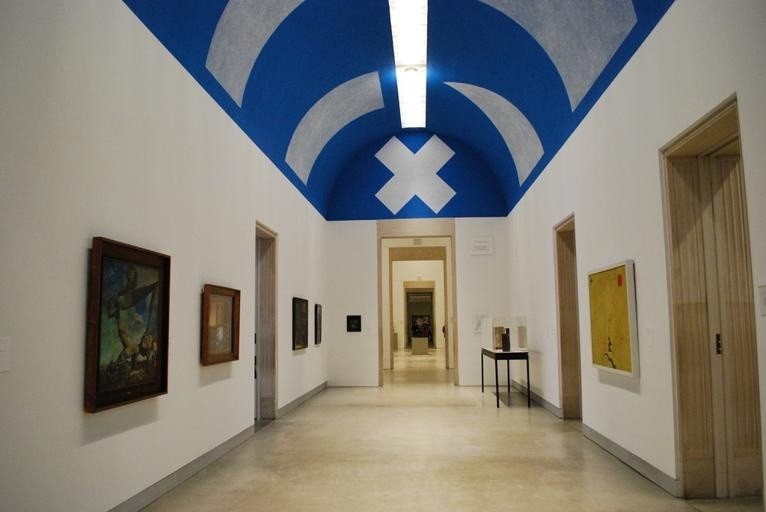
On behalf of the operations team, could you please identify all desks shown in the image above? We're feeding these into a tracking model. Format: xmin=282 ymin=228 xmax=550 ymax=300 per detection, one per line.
xmin=480 ymin=346 xmax=531 ymax=411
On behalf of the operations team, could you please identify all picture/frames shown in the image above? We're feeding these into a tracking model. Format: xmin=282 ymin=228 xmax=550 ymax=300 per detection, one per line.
xmin=314 ymin=303 xmax=322 ymax=345
xmin=291 ymin=296 xmax=309 ymax=350
xmin=347 ymin=315 xmax=361 ymax=332
xmin=83 ymin=236 xmax=171 ymax=414
xmin=586 ymin=259 xmax=641 ymax=378
xmin=200 ymin=283 xmax=242 ymax=366
